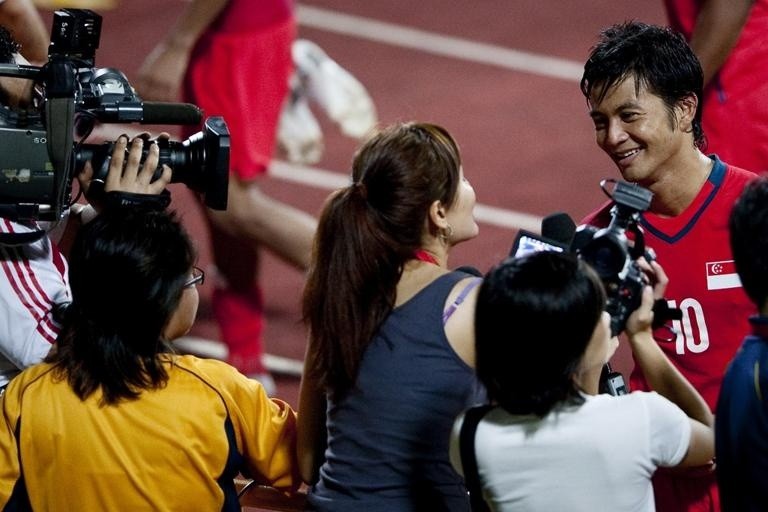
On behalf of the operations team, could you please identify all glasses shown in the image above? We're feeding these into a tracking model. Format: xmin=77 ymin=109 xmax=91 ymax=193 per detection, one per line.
xmin=182 ymin=266 xmax=205 ymax=287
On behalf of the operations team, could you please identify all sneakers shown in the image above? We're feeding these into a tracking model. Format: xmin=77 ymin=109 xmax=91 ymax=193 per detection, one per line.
xmin=276 ymin=39 xmax=377 ymax=164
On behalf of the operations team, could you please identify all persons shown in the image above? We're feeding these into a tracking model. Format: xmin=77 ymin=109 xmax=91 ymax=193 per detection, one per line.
xmin=296 ymin=121 xmax=484 ymax=512
xmin=576 ymin=1 xmax=768 ymax=512
xmin=0 ymin=0 xmax=379 ymax=510
xmin=449 ymin=244 xmax=717 ymax=511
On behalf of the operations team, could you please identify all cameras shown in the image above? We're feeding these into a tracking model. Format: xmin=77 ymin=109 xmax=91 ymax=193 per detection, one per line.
xmin=507 ymin=179 xmax=682 ymax=339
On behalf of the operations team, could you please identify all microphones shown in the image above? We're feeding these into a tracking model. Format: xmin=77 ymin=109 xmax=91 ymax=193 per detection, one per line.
xmin=538 ymin=213 xmax=576 ymax=244
